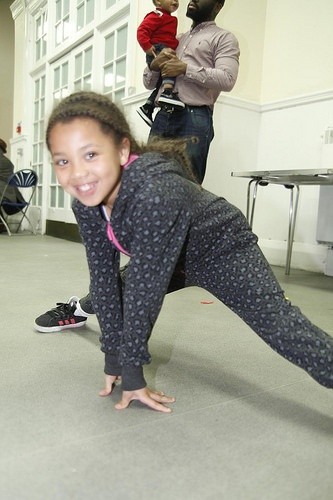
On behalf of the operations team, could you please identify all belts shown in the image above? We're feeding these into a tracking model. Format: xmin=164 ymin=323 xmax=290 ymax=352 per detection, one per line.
xmin=164 ymin=104 xmax=180 ymax=113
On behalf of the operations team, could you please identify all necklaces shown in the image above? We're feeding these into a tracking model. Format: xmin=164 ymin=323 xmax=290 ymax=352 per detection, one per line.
xmin=32 ymin=91 xmax=333 ymax=412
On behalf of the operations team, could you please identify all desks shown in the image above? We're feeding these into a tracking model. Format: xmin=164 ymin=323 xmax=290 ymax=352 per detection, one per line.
xmin=232 ymin=169 xmax=333 ymax=276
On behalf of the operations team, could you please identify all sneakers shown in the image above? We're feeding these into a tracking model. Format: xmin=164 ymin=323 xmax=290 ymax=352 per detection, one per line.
xmin=158 ymin=91 xmax=186 ymax=109
xmin=34 ymin=295 xmax=88 ymax=333
xmin=137 ymin=105 xmax=154 ymax=127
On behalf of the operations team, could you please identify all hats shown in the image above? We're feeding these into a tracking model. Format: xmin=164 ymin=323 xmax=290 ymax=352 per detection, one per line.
xmin=45 ymin=90 xmax=133 ymax=146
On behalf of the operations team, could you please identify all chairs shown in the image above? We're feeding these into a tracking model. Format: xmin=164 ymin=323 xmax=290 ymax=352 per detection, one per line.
xmin=0 ymin=169 xmax=38 ymax=236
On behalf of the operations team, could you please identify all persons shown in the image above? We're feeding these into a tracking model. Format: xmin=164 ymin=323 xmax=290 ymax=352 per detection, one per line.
xmin=0 ymin=139 xmax=25 ymax=233
xmin=141 ymin=0 xmax=240 ymax=185
xmin=136 ymin=0 xmax=185 ymax=126
